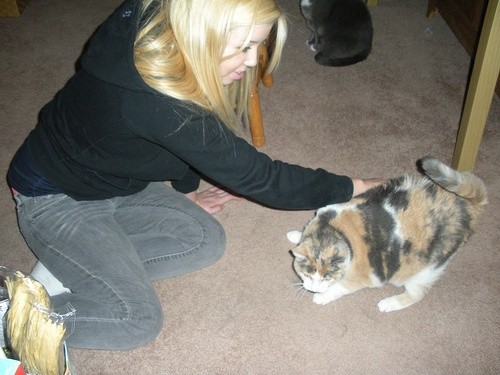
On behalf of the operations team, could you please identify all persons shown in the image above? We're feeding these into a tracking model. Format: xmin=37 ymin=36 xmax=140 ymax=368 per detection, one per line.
xmin=1 ymin=0 xmax=391 ymax=351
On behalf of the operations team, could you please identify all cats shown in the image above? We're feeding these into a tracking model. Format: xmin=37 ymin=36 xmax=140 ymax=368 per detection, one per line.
xmin=301 ymin=0 xmax=373 ymax=67
xmin=286 ymin=155 xmax=488 ymax=312
